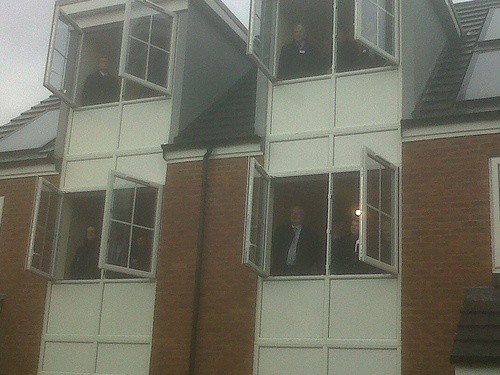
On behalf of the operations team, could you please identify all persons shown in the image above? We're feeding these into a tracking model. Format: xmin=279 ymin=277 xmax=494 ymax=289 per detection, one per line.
xmin=278 ymin=24 xmax=319 ymax=81
xmin=70 ymin=225 xmax=152 ymax=280
xmin=271 ymin=205 xmax=314 ymax=277
xmin=331 ymin=217 xmax=391 ymax=274
xmin=84 ymin=57 xmax=120 ymax=106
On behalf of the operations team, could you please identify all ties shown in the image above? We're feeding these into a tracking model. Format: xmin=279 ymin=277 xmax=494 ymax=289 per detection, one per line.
xmin=110 ymin=245 xmax=116 ymax=265
xmin=298 ymin=44 xmax=301 ymax=49
